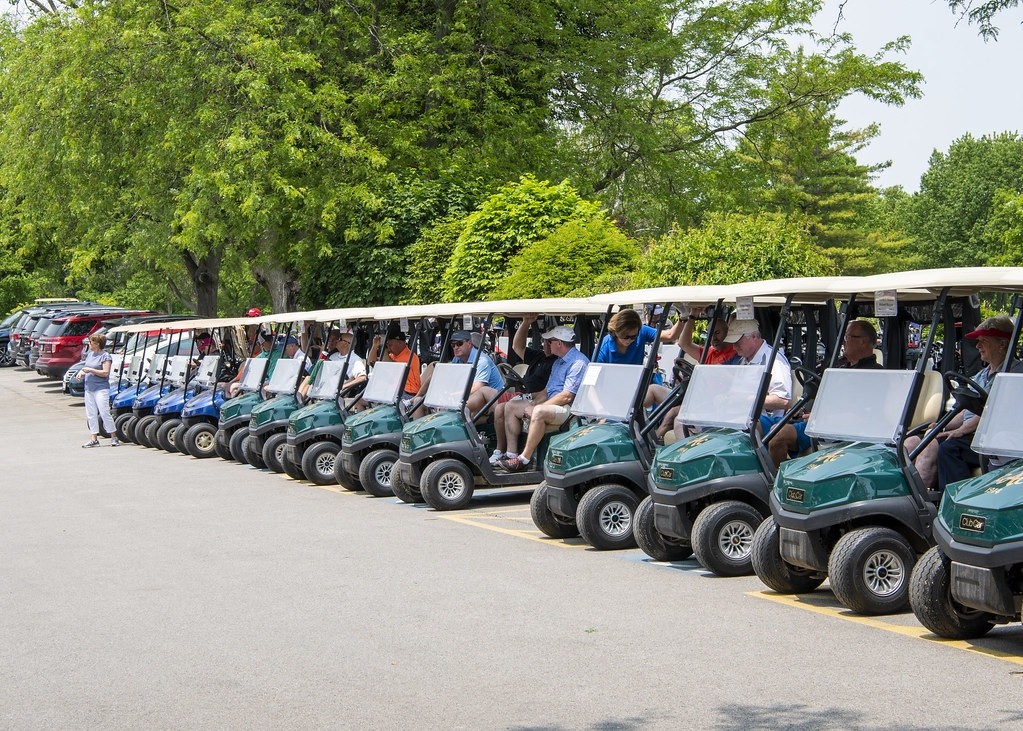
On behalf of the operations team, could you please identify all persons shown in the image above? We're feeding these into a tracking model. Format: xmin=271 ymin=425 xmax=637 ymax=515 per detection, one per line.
xmin=592 ymin=307 xmax=1022 ymax=508
xmin=495 ymin=325 xmax=591 ymax=473
xmin=190 ymin=312 xmax=558 ymax=465
xmin=75 ymin=334 xmax=120 ymax=448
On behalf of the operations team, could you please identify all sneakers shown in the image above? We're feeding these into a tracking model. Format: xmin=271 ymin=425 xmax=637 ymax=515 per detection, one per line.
xmin=495 ymin=455 xmax=510 ymax=461
xmin=489 ymin=449 xmax=503 ymax=464
xmin=111 ymin=437 xmax=120 ymax=446
xmin=82 ymin=440 xmax=100 ymax=447
xmin=498 ymin=457 xmax=531 ymax=473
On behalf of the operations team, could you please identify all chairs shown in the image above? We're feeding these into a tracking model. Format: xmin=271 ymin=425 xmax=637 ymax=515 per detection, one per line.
xmin=910 ymin=369 xmax=943 ymax=430
xmin=788 ymin=370 xmax=808 ymax=415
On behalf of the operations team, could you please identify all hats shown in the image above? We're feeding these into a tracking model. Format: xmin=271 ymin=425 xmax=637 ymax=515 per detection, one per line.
xmin=448 ymin=331 xmax=471 ymax=341
xmin=276 ymin=336 xmax=298 ymax=348
xmin=258 ymin=331 xmax=274 ymax=346
xmin=542 ymin=326 xmax=576 ymax=342
xmin=387 ymin=331 xmax=407 ymax=341
xmin=246 ymin=307 xmax=262 ymax=316
xmin=195 ymin=332 xmax=210 ymax=343
xmin=965 ymin=325 xmax=1012 ymax=339
xmin=722 ymin=318 xmax=759 ymax=343
xmin=197 ymin=338 xmax=215 ymax=350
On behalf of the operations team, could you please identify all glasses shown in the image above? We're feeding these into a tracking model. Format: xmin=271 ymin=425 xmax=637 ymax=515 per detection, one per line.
xmin=338 ymin=337 xmax=351 ymax=345
xmin=548 ymin=339 xmax=560 ymax=344
xmin=621 ymin=330 xmax=640 ymax=341
xmin=844 ymin=335 xmax=863 ymax=341
xmin=450 ymin=340 xmax=468 ymax=347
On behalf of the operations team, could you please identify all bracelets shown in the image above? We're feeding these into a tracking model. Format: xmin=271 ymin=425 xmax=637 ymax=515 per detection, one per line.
xmin=679 ymin=318 xmax=688 ymax=323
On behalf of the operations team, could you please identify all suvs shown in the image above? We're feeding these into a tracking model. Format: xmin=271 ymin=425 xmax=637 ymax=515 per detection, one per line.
xmin=0 ymin=297 xmax=207 ymax=398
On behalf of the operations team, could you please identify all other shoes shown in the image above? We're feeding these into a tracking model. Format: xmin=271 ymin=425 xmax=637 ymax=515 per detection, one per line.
xmin=402 ymin=399 xmax=412 ymax=408
